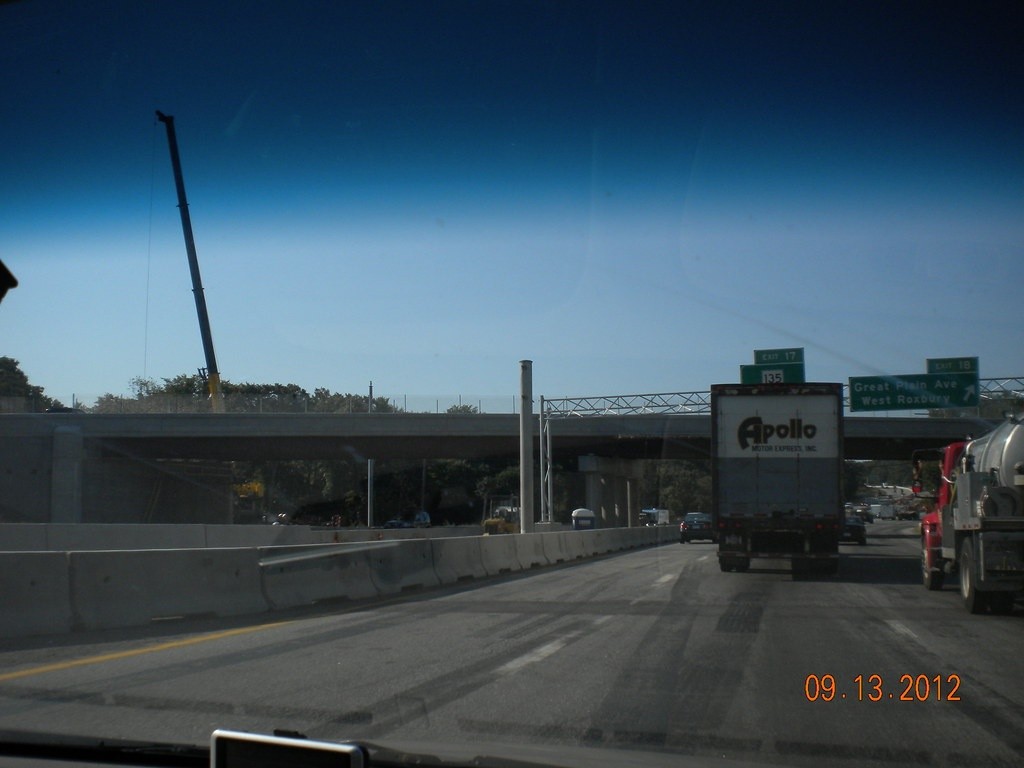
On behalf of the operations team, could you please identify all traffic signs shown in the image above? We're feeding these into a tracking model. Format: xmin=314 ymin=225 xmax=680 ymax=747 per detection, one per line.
xmin=848 ymin=355 xmax=982 ymax=413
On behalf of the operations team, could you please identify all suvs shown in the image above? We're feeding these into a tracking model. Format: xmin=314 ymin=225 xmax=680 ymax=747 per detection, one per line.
xmin=384 ymin=509 xmax=431 ymax=530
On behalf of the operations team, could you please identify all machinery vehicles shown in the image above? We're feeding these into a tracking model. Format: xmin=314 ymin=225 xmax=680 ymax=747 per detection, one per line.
xmin=153 ymin=107 xmax=266 ymax=529
xmin=480 ymin=491 xmax=520 ymax=535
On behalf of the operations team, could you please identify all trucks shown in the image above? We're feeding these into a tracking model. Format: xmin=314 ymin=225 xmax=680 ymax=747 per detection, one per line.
xmin=906 ymin=411 xmax=1024 ymax=617
xmin=708 ymin=380 xmax=848 ymax=578
xmin=638 ymin=507 xmax=670 ymax=527
xmin=571 ymin=508 xmax=595 ymax=531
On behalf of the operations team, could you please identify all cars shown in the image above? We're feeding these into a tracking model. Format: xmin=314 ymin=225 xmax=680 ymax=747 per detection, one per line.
xmin=43 ymin=407 xmax=86 ymax=414
xmin=839 ymin=491 xmax=931 ymax=546
xmin=679 ymin=512 xmax=720 ymax=546
